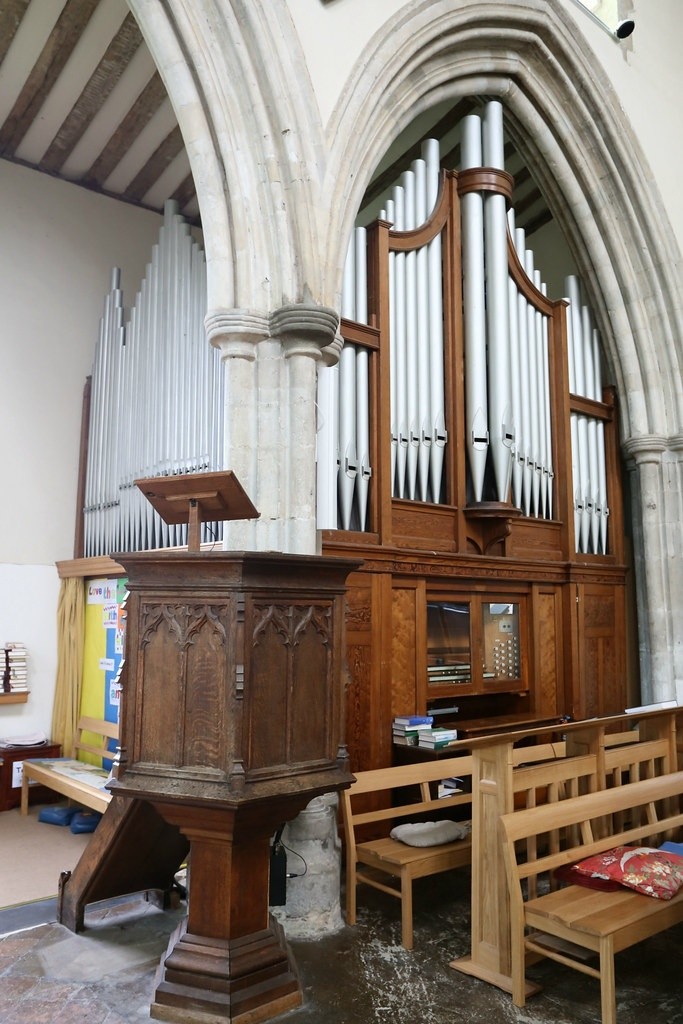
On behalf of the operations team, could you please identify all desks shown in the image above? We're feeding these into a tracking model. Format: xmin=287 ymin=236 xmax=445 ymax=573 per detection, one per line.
xmin=0 ymin=738 xmax=62 ymax=812
xmin=390 ymin=711 xmax=563 ymax=838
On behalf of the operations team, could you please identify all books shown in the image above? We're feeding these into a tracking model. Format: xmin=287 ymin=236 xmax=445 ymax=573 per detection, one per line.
xmin=392 ymin=716 xmax=433 ymax=746
xmin=441 ymin=779 xmax=461 ymax=788
xmin=417 ymin=727 xmax=457 ymax=750
xmin=4 ymin=642 xmax=31 ymax=692
xmin=0 ymin=649 xmax=7 ymax=693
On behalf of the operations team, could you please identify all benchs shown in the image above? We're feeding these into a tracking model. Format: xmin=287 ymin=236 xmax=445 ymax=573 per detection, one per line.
xmin=20 ymin=715 xmax=120 ymax=818
xmin=341 ymin=727 xmax=683 ymax=1024
